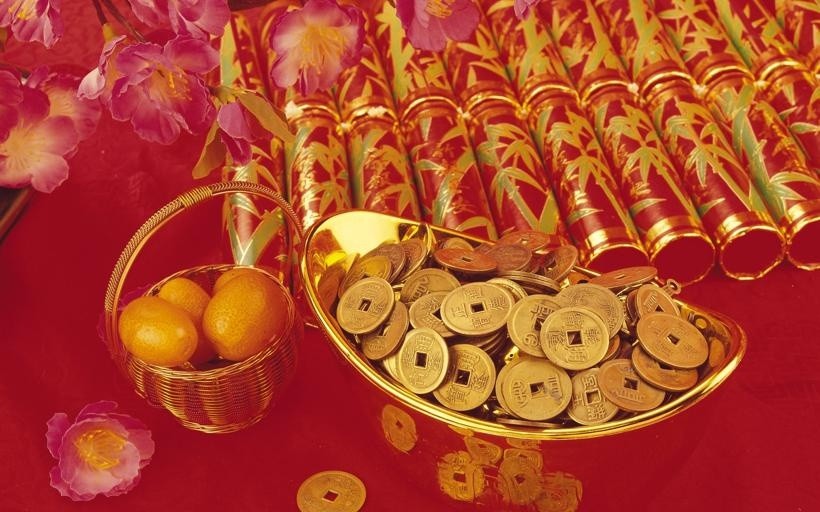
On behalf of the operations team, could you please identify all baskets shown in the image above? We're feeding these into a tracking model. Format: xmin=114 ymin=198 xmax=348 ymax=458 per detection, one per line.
xmin=104 ymin=181 xmax=304 ymax=435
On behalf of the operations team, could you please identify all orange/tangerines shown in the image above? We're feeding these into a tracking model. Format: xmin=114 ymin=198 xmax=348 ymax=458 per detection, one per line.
xmin=118 ymin=269 xmax=288 ymax=368
